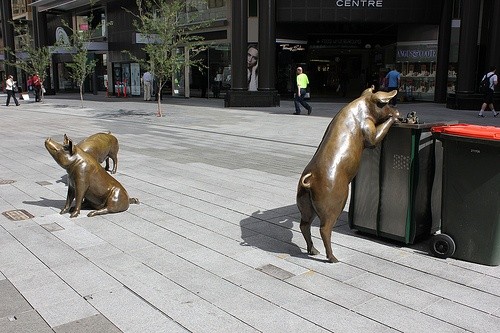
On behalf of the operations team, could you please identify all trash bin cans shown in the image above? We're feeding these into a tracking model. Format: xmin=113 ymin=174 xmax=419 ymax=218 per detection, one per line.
xmin=430 ymin=121 xmax=500 ymax=268
xmin=347 ymin=113 xmax=458 ymax=246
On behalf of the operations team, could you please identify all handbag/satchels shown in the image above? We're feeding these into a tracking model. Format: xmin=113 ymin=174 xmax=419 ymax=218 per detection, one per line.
xmin=304 ymin=91 xmax=310 ymax=98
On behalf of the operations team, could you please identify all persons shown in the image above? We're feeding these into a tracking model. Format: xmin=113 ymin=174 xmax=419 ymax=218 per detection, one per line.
xmin=201 ymin=65 xmax=222 ymax=98
xmin=478 ymin=65 xmax=500 ymax=118
xmin=293 ymin=66 xmax=312 ymax=115
xmin=142 ymin=71 xmax=163 ymax=102
xmin=248 ymin=44 xmax=260 ymax=91
xmin=6 ymin=72 xmax=43 ymax=106
xmin=114 ymin=65 xmax=140 ymax=86
xmin=382 ymin=66 xmax=401 ymax=107
xmin=57 ymin=29 xmax=69 ymax=46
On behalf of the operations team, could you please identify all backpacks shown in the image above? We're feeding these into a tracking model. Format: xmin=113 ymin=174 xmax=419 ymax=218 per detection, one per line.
xmin=481 ymin=74 xmax=496 ymax=91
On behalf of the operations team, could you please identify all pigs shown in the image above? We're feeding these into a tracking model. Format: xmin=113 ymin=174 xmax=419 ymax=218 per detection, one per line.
xmin=45 ymin=133 xmax=138 ymax=218
xmin=76 ymin=131 xmax=119 ymax=174
xmin=297 ymin=84 xmax=400 ymax=264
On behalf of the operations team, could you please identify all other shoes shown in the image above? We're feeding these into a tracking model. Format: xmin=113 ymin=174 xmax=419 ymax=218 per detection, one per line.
xmin=144 ymin=100 xmax=147 ymax=101
xmin=493 ymin=112 xmax=500 ymax=117
xmin=16 ymin=104 xmax=20 ymax=106
xmin=293 ymin=112 xmax=301 ymax=115
xmin=6 ymin=105 xmax=10 ymax=106
xmin=147 ymin=99 xmax=152 ymax=101
xmin=307 ymin=105 xmax=313 ymax=115
xmin=478 ymin=115 xmax=485 ymax=117
